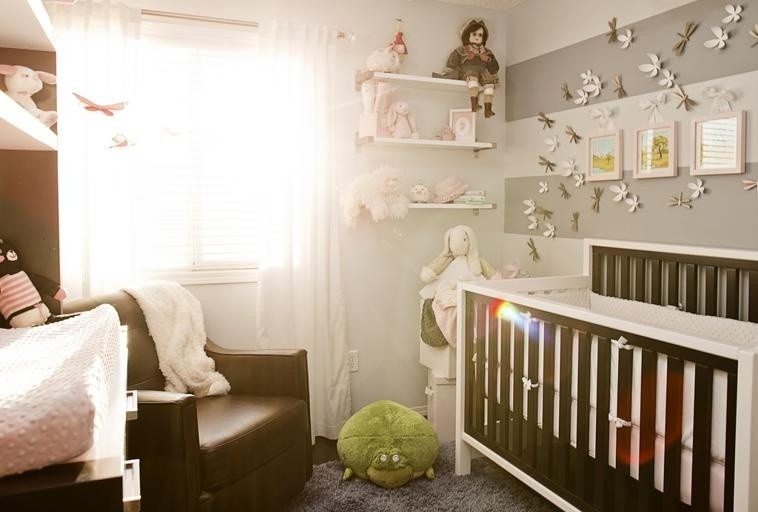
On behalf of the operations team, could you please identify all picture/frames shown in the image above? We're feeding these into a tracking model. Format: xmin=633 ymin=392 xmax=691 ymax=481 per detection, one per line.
xmin=690 ymin=110 xmax=746 ymax=176
xmin=445 ymin=106 xmax=477 ymax=143
xmin=632 ymin=121 xmax=677 ymax=179
xmin=585 ymin=128 xmax=622 ymax=181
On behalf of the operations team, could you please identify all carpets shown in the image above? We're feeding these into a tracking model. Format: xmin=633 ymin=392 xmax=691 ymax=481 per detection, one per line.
xmin=293 ymin=439 xmax=560 ymax=511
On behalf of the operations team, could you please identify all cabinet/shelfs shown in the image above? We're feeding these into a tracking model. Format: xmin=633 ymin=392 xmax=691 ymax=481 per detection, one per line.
xmin=352 ymin=65 xmax=497 ymax=218
xmin=0 ymin=1 xmax=60 ymax=330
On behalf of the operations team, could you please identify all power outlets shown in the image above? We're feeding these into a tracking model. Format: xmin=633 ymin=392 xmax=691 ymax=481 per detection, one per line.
xmin=342 ymin=349 xmax=362 ymax=374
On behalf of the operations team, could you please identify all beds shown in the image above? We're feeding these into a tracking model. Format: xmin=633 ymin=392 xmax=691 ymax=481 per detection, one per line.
xmin=454 ymin=237 xmax=758 ymax=512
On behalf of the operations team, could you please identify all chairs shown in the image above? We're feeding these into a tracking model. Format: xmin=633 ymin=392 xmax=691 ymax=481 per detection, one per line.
xmin=62 ymin=283 xmax=314 ymax=512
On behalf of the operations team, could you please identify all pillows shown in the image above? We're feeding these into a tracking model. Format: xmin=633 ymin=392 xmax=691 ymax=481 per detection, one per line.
xmin=0 ymin=303 xmax=121 ymax=480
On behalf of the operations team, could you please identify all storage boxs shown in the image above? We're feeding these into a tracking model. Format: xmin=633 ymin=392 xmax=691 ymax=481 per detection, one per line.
xmin=419 ymin=337 xmax=456 ymax=379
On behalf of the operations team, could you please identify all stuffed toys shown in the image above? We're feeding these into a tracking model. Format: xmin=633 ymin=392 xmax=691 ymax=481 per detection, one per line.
xmin=0 ymin=236 xmax=82 ymax=329
xmin=443 ymin=124 xmax=456 ymax=142
xmin=336 ymin=399 xmax=441 ymax=483
xmin=0 ymin=62 xmax=58 ymax=130
xmin=360 ymin=41 xmax=403 ymax=118
xmin=342 ymin=168 xmax=411 ymax=229
xmin=383 ymin=98 xmax=421 ymax=140
xmin=410 ymin=180 xmax=434 ymax=204
xmin=419 ymin=225 xmax=504 ymax=349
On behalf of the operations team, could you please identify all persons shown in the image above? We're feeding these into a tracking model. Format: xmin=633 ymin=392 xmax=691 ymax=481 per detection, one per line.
xmin=440 ymin=15 xmax=500 ymax=120
